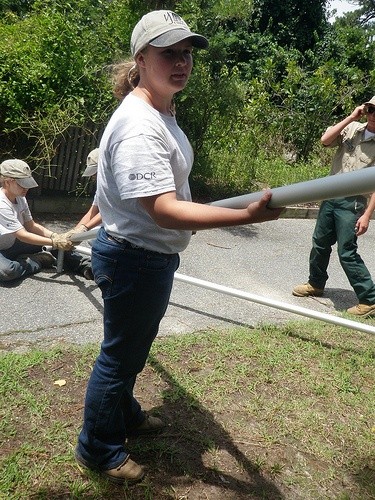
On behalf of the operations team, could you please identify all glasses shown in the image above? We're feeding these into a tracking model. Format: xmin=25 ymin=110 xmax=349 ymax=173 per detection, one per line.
xmin=367 ymin=106 xmax=375 ymax=113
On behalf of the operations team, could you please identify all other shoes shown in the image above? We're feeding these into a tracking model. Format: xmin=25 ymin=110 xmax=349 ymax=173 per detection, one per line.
xmin=347 ymin=303 xmax=375 ymax=319
xmin=292 ymin=281 xmax=324 ymax=297
xmin=127 ymin=416 xmax=162 ymax=431
xmin=78 ymin=258 xmax=93 ymax=279
xmin=107 ymin=454 xmax=144 ymax=480
xmin=31 ymin=252 xmax=55 ymax=267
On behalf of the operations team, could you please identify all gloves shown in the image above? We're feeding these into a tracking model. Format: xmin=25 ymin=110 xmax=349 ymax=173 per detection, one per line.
xmin=51 ymin=233 xmax=72 ymax=251
xmin=64 ymin=224 xmax=89 ymax=245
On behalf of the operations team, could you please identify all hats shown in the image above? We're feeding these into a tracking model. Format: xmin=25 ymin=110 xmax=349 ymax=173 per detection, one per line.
xmin=0 ymin=158 xmax=39 ymax=188
xmin=364 ymin=96 xmax=375 ymax=105
xmin=81 ymin=148 xmax=98 ymax=177
xmin=130 ymin=10 xmax=210 ymax=58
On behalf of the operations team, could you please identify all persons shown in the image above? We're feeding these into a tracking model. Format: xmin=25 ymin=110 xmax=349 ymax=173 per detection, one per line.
xmin=65 ymin=148 xmax=102 ymax=245
xmin=292 ymin=95 xmax=375 ymax=317
xmin=0 ymin=147 xmax=93 ymax=280
xmin=73 ymin=10 xmax=286 ymax=484
